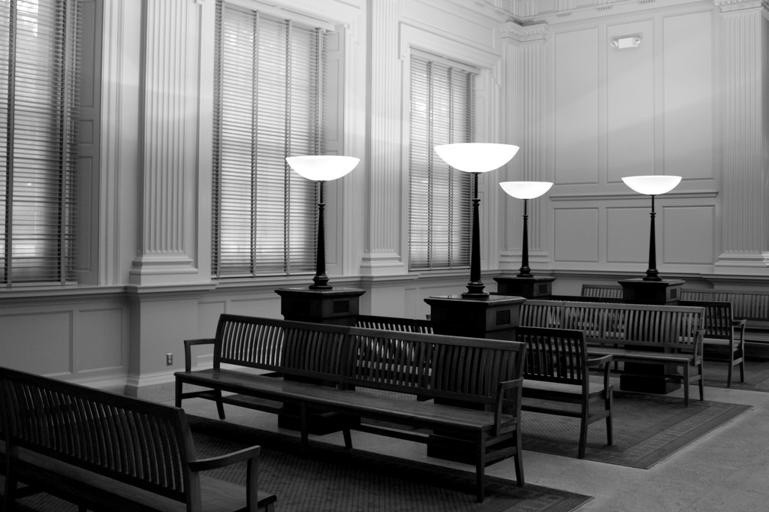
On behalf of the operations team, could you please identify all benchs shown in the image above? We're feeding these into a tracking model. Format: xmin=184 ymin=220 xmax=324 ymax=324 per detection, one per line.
xmin=487 ymin=290 xmax=745 ymax=392
xmin=424 ymin=294 xmax=708 ymax=410
xmin=170 ymin=311 xmax=530 ymax=506
xmin=578 ymin=284 xmax=760 ymax=346
xmin=342 ymin=314 xmax=618 ymax=462
xmin=3 ymin=360 xmax=281 ymax=509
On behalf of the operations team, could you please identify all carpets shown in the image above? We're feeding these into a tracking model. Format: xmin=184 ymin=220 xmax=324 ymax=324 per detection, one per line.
xmin=558 ymin=341 xmax=767 ymax=394
xmin=338 ymin=365 xmax=756 ymax=475
xmin=44 ymin=401 xmax=598 ymax=510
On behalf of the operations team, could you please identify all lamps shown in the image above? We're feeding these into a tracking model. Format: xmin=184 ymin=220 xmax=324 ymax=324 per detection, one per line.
xmin=496 ymin=180 xmax=555 ymax=278
xmin=621 ymin=174 xmax=683 ymax=282
xmin=284 ymin=154 xmax=362 ymax=292
xmin=434 ymin=142 xmax=521 ymax=300
